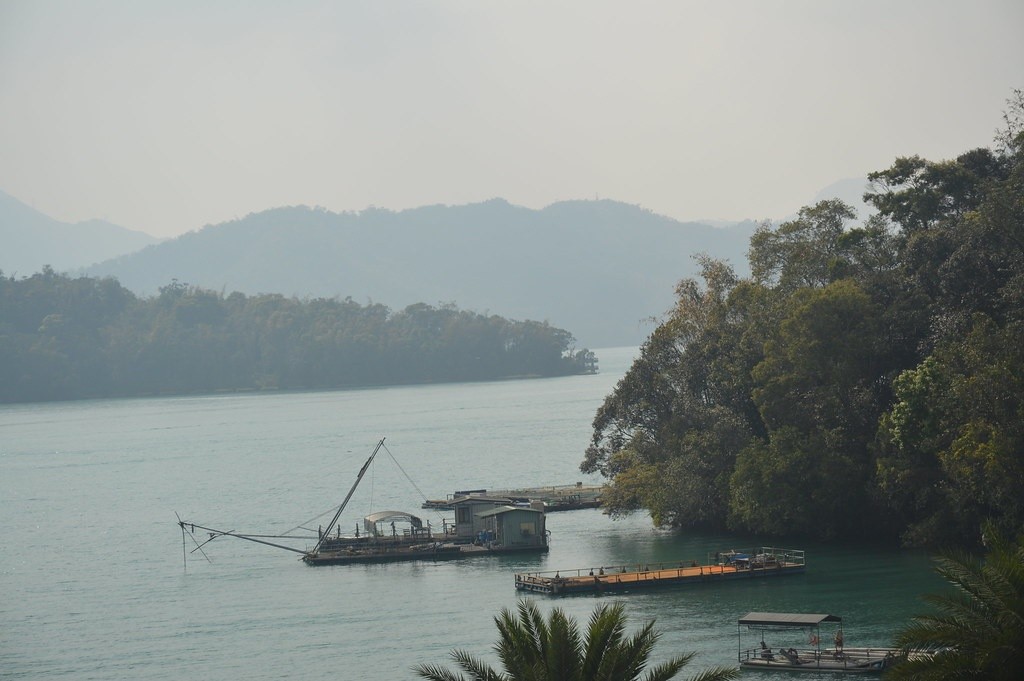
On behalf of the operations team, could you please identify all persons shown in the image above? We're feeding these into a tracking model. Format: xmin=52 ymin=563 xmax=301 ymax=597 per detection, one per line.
xmin=836 ymin=630 xmax=844 ymax=651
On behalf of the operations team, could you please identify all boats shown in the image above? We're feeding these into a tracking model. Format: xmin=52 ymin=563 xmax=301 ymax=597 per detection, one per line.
xmin=300 ymin=435 xmax=552 ymax=564
xmin=738 ymin=612 xmax=908 ymax=674
